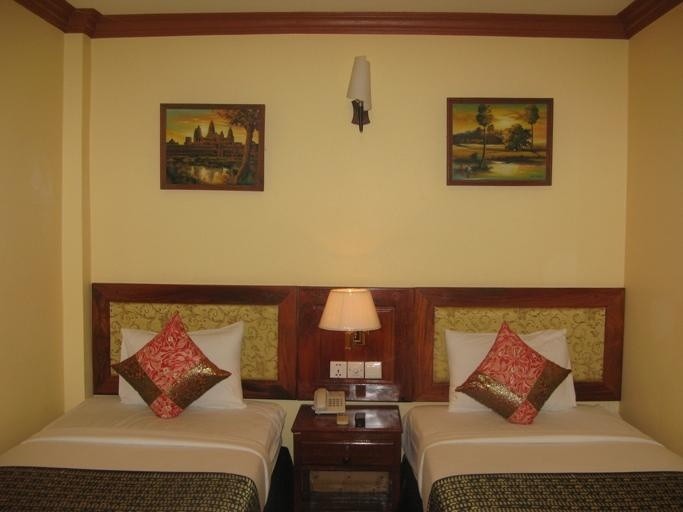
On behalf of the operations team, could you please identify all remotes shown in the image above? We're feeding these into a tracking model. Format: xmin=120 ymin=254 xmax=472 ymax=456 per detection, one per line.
xmin=355 ymin=412 xmax=365 ymax=427
xmin=337 ymin=412 xmax=349 ymax=425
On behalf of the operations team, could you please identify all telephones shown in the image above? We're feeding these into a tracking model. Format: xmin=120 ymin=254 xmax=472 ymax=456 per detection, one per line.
xmin=314 ymin=388 xmax=346 ymax=414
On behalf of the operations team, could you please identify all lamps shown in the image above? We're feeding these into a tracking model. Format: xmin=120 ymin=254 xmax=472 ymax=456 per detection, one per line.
xmin=318 ymin=288 xmax=383 ymax=349
xmin=347 ymin=55 xmax=372 ymax=132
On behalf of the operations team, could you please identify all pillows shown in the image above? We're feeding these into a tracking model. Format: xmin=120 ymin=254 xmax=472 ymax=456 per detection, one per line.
xmin=455 ymin=321 xmax=572 ymax=425
xmin=445 ymin=329 xmax=577 ymax=412
xmin=109 ymin=311 xmax=232 ymax=418
xmin=119 ymin=321 xmax=246 ymax=411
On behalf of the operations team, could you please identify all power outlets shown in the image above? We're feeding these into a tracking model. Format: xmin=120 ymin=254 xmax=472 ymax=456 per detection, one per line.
xmin=329 ymin=361 xmax=347 ymax=379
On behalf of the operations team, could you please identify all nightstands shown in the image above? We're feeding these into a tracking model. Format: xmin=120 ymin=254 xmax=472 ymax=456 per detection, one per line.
xmin=291 ymin=405 xmax=402 ymax=512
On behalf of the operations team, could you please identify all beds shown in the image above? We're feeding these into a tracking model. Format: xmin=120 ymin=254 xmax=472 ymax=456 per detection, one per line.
xmin=401 ymin=286 xmax=683 ymax=512
xmin=0 ymin=283 xmax=299 ymax=511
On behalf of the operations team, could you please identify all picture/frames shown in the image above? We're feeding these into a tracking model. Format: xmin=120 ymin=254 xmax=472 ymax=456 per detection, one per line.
xmin=160 ymin=103 xmax=265 ymax=192
xmin=446 ymin=97 xmax=553 ymax=186
